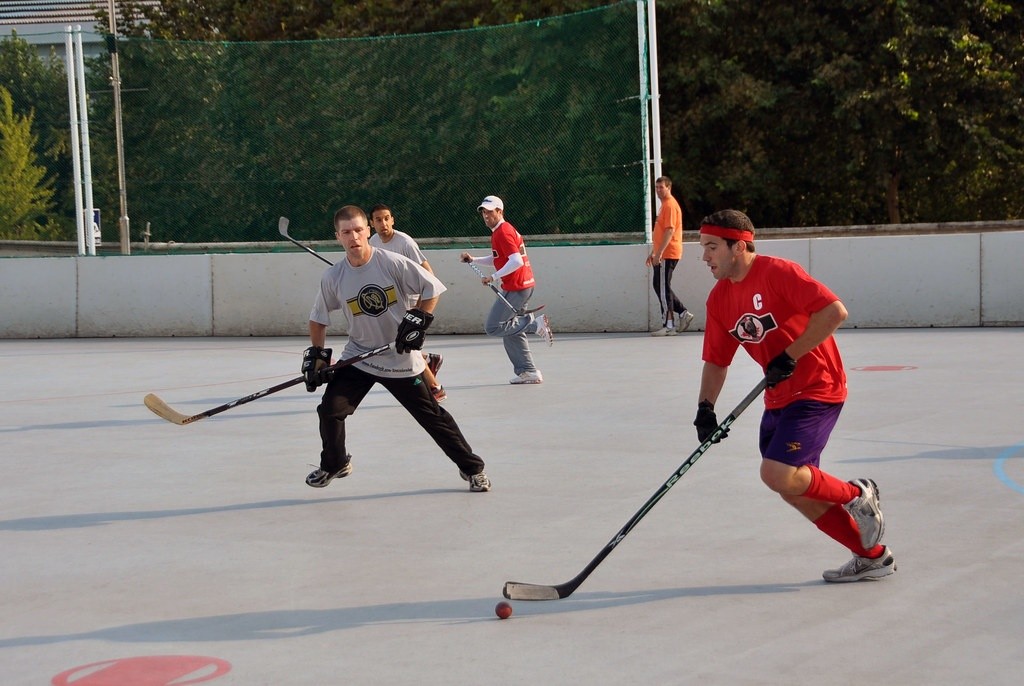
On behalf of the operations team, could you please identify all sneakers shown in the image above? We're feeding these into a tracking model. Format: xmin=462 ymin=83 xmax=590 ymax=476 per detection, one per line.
xmin=842 ymin=477 xmax=885 ymax=549
xmin=677 ymin=311 xmax=693 ymax=334
xmin=534 ymin=313 xmax=553 ymax=347
xmin=306 ymin=453 xmax=352 ymax=488
xmin=459 ymin=467 xmax=492 ymax=492
xmin=822 ymin=544 xmax=896 ymax=582
xmin=509 ymin=368 xmax=542 ymax=384
xmin=651 ymin=327 xmax=677 ymax=336
xmin=425 ymin=351 xmax=443 ymax=377
xmin=430 ymin=383 xmax=446 ymax=403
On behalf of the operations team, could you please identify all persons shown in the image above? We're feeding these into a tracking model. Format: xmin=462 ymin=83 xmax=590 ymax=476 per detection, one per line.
xmin=460 ymin=196 xmax=553 ymax=384
xmin=645 ymin=176 xmax=694 ymax=336
xmin=368 ymin=205 xmax=447 ymax=403
xmin=301 ymin=206 xmax=491 ymax=492
xmin=694 ymin=210 xmax=897 ymax=585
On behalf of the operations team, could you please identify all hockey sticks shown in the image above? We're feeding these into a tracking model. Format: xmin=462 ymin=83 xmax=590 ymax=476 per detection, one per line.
xmin=503 ymin=378 xmax=766 ymax=600
xmin=143 ymin=341 xmax=395 ymax=425
xmin=464 ymin=257 xmax=546 ymax=316
xmin=279 ymin=216 xmax=334 ymax=267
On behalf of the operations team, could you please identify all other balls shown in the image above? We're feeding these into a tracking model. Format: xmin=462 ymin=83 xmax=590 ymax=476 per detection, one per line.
xmin=495 ymin=602 xmax=513 ymax=619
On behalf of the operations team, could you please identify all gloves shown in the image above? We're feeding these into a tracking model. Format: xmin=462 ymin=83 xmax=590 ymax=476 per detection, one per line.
xmin=395 ymin=307 xmax=434 ymax=353
xmin=301 ymin=346 xmax=332 ymax=392
xmin=694 ymin=399 xmax=728 ymax=446
xmin=766 ymin=350 xmax=797 ymax=388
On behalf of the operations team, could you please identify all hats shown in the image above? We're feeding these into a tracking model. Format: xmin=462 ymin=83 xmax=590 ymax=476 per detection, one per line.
xmin=477 ymin=195 xmax=504 ymax=211
